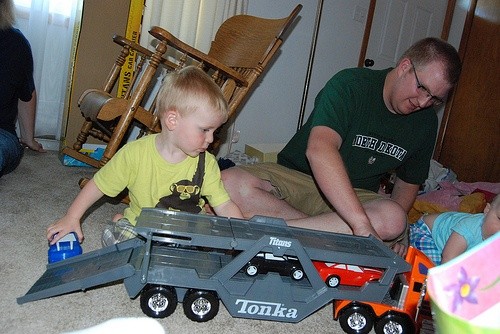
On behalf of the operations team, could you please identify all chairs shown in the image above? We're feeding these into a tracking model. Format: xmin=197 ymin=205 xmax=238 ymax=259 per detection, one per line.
xmin=62 ymin=4 xmax=303 ymax=205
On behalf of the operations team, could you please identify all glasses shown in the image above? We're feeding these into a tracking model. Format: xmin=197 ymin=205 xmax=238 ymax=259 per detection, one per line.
xmin=407 ymin=58 xmax=445 ymax=106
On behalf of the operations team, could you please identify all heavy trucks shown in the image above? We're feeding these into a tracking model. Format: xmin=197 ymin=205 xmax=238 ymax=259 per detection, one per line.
xmin=16 ymin=206 xmax=435 ymax=334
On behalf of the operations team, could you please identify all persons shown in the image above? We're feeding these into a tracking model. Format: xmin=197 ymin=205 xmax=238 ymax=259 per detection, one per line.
xmin=423 ymin=193 xmax=500 ymax=267
xmin=205 ymin=37 xmax=461 ymax=258
xmin=0 ymin=0 xmax=47 ymax=177
xmin=45 ymin=66 xmax=245 ymax=249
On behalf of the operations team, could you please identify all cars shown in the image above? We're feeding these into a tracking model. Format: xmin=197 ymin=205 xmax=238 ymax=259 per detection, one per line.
xmin=231 ymin=250 xmax=305 ymax=282
xmin=47 ymin=231 xmax=82 ymax=264
xmin=312 ymin=261 xmax=384 ymax=288
